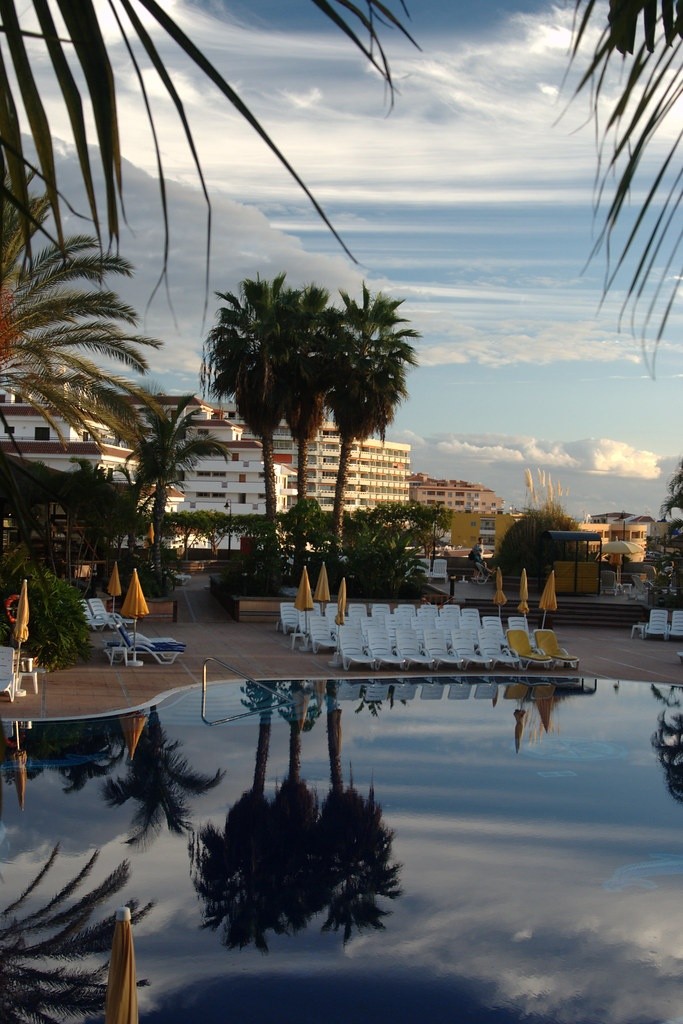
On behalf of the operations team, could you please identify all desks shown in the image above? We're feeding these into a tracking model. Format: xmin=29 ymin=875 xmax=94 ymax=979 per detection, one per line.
xmin=18 ymin=671 xmax=38 ymax=695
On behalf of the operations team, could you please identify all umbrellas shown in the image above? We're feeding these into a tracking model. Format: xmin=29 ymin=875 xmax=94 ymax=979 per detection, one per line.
xmin=294 ymin=566 xmax=314 ymax=648
xmin=598 ymin=536 xmax=644 ymax=584
xmin=10 ymin=721 xmax=28 ymax=811
xmin=292 ymin=680 xmax=311 ymax=732
xmin=119 ymin=712 xmax=147 ymax=760
xmin=314 ymin=680 xmax=327 ymax=714
xmin=314 ymin=562 xmax=331 ymax=614
xmin=517 ymin=568 xmax=529 ymax=630
xmin=104 ymin=906 xmax=138 ymax=1024
xmin=144 ymin=523 xmax=155 ymax=548
xmin=335 ymin=577 xmax=346 ymax=662
xmin=539 ymin=570 xmax=557 ymax=629
xmin=13 ymin=579 xmax=29 ymax=697
xmin=120 ymin=568 xmax=150 ymax=666
xmin=329 ymin=703 xmax=342 ymax=754
xmin=106 ymin=562 xmax=121 ymax=613
xmin=493 ymin=566 xmax=507 ymax=617
xmin=513 ymin=697 xmax=553 ymax=752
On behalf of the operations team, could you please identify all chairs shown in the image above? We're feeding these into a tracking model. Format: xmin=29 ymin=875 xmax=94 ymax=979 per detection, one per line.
xmin=1 ymin=720 xmax=14 ymax=738
xmin=630 ymin=610 xmax=683 ymax=641
xmin=600 ymin=570 xmax=650 ymax=601
xmin=275 ymin=602 xmax=580 ymax=671
xmin=77 ymin=599 xmax=185 ymax=666
xmin=414 ymin=558 xmax=448 ymax=583
xmin=0 ymin=647 xmax=17 ymax=703
xmin=337 ymin=683 xmax=559 ymax=701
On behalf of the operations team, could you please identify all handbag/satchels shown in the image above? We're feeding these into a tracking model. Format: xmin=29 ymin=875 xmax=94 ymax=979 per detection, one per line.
xmin=469 ymin=551 xmax=475 ymax=560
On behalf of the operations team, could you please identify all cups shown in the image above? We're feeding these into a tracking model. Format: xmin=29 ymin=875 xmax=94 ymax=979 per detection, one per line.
xmin=27 ymin=658 xmax=33 ymax=673
xmin=20 ymin=658 xmax=27 ymax=672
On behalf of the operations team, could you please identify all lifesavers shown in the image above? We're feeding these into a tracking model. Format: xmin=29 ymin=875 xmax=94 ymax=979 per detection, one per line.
xmin=5 ymin=594 xmax=21 ymax=622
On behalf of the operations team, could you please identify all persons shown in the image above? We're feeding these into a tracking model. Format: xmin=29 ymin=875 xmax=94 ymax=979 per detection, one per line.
xmin=472 ymin=537 xmax=493 ymax=574
